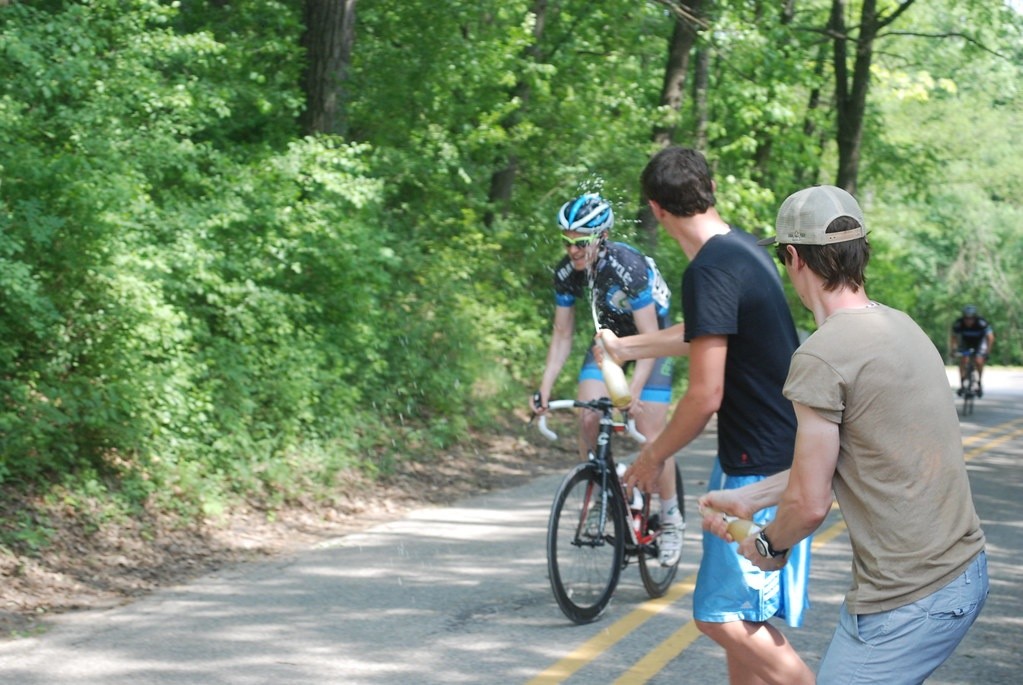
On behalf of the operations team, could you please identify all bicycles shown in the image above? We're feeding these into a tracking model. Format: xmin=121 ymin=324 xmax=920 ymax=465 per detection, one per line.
xmin=950 ymin=348 xmax=990 ymax=417
xmin=525 ymin=387 xmax=686 ymax=626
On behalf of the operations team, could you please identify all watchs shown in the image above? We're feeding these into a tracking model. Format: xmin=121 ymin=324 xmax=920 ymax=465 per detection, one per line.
xmin=752 ymin=527 xmax=789 ymax=560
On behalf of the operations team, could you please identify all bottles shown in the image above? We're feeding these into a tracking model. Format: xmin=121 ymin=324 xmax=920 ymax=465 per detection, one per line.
xmin=615 ymin=462 xmax=635 ymax=505
xmin=698 ymin=506 xmax=790 ymax=568
xmin=594 ymin=334 xmax=632 ymax=405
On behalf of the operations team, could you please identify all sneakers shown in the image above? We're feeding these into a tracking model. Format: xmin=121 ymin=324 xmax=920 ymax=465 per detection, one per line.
xmin=656 ymin=510 xmax=685 ymax=566
xmin=580 ymin=497 xmax=611 ymax=544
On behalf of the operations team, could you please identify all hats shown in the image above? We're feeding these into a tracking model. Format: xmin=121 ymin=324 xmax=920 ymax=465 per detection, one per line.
xmin=757 ymin=183 xmax=871 ymax=246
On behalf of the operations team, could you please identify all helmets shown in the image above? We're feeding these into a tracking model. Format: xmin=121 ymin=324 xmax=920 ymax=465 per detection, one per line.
xmin=558 ymin=194 xmax=614 ymax=233
xmin=962 ymin=303 xmax=979 ymax=316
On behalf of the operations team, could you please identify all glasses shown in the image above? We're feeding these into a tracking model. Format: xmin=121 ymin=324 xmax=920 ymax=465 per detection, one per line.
xmin=964 ymin=316 xmax=976 ymax=320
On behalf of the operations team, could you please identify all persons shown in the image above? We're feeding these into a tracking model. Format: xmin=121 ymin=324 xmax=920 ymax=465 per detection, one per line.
xmin=592 ymin=145 xmax=819 ymax=685
xmin=698 ymin=180 xmax=990 ymax=684
xmin=950 ymin=307 xmax=996 ymax=400
xmin=526 ymin=192 xmax=686 ymax=569
